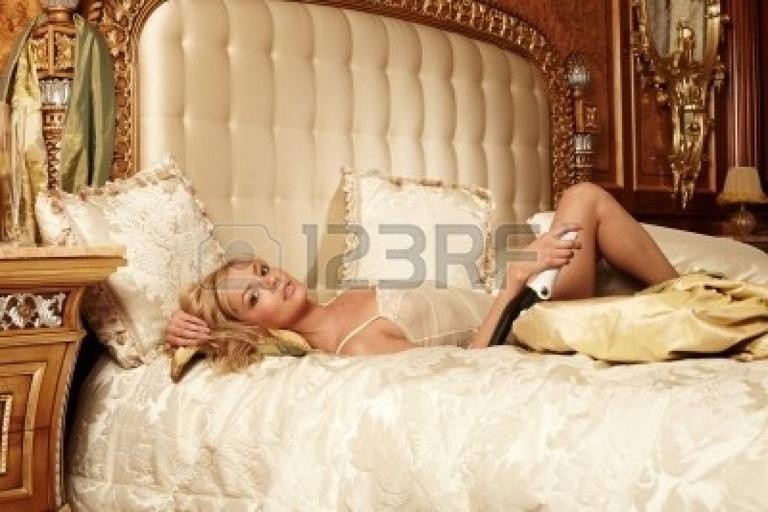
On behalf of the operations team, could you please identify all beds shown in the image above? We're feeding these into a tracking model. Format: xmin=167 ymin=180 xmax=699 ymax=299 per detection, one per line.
xmin=29 ymin=0 xmax=768 ymax=508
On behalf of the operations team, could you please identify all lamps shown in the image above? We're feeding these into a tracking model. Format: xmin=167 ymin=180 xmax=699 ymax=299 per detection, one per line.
xmin=716 ymin=169 xmax=767 ymax=232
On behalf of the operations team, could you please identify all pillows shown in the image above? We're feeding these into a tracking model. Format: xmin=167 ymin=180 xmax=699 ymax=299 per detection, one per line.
xmin=38 ymin=159 xmax=228 ymax=366
xmin=324 ymin=162 xmax=490 ymax=302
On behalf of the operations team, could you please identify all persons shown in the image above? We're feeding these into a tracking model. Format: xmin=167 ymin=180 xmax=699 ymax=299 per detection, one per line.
xmin=165 ymin=183 xmax=683 ymax=375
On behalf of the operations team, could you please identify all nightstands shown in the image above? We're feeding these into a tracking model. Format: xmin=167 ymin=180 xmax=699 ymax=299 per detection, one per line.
xmin=0 ymin=245 xmax=124 ymax=510
